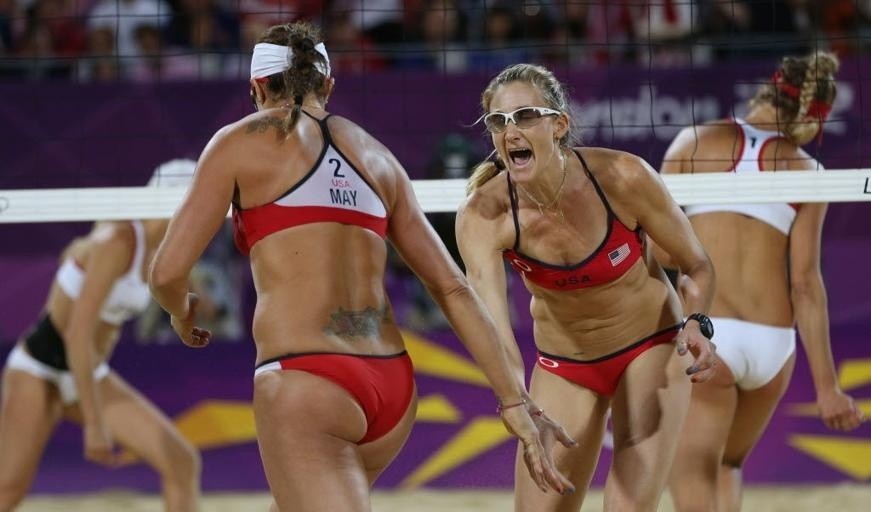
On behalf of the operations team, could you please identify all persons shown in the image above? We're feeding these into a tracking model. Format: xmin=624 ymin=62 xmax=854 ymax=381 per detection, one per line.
xmin=455 ymin=62 xmax=720 ymax=512
xmin=149 ymin=24 xmax=564 ymax=512
xmin=646 ymin=51 xmax=868 ymax=512
xmin=0 ymin=160 xmax=198 ymax=512
xmin=0 ymin=0 xmax=871 ymax=83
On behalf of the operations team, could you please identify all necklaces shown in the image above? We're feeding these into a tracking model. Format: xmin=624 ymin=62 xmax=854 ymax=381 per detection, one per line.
xmin=511 ymin=168 xmax=566 ymax=220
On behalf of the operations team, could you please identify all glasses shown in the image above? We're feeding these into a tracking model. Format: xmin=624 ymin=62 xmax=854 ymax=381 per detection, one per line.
xmin=483 ymin=107 xmax=560 ymax=134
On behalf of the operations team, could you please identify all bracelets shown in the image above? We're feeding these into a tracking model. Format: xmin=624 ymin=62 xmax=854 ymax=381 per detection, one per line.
xmin=530 ymin=409 xmax=544 ymax=417
xmin=495 ymin=400 xmax=527 ymax=418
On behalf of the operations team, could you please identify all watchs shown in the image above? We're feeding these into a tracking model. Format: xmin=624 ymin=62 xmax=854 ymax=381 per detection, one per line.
xmin=682 ymin=313 xmax=714 ymax=340
